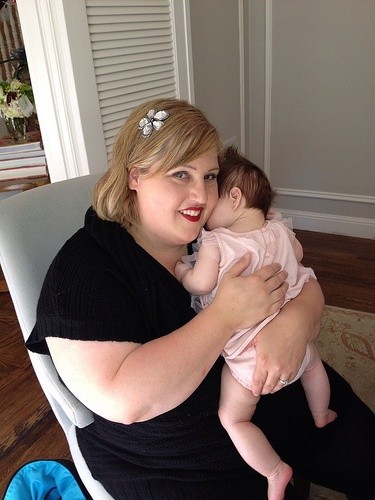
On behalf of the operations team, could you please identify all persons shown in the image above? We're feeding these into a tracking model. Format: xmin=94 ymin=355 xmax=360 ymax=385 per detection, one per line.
xmin=174 ymin=143 xmax=337 ymax=500
xmin=24 ymin=99 xmax=374 ymax=500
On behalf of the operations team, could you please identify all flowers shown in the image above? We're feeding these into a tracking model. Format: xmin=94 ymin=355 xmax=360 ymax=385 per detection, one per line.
xmin=138 ymin=108 xmax=170 ymax=138
xmin=0 ymin=77 xmax=37 ymax=137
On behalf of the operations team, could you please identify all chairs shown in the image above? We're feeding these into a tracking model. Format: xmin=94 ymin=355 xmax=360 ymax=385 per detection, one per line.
xmin=0 ymin=170 xmax=114 ymax=500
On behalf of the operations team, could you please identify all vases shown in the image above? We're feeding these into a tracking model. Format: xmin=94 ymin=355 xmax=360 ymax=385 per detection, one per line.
xmin=3 ymin=112 xmax=26 ymax=142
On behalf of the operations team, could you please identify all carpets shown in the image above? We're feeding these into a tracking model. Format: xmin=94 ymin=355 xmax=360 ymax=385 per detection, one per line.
xmin=309 ymin=305 xmax=375 ymax=500
